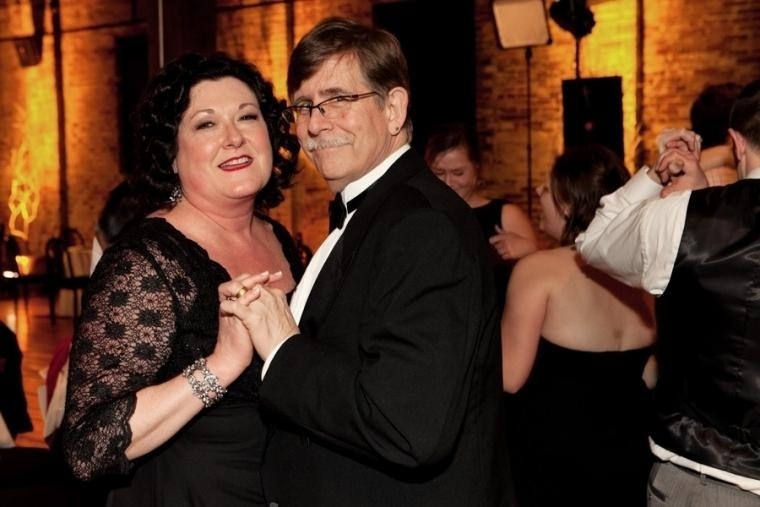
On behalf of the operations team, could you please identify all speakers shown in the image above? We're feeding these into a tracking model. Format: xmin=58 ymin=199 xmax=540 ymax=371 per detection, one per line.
xmin=560 ymin=76 xmax=626 ymax=162
xmin=548 ymin=0 xmax=595 ymax=38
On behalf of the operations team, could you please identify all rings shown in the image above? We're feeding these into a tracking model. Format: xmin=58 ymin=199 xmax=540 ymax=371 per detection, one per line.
xmin=236 ymin=286 xmax=248 ymax=297
xmin=227 ymin=296 xmax=238 ymax=301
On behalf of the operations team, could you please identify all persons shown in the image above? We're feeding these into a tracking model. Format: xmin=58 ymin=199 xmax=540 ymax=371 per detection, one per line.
xmin=423 ymin=119 xmax=539 ymax=317
xmin=573 ymin=77 xmax=759 ymax=506
xmin=214 ymin=15 xmax=512 ymax=505
xmin=54 ymin=49 xmax=305 ymax=506
xmin=686 ymin=81 xmax=745 ymax=190
xmin=42 ymin=175 xmax=178 ymax=449
xmin=654 ymin=137 xmax=710 ymax=198
xmin=500 ymin=142 xmax=664 ymax=506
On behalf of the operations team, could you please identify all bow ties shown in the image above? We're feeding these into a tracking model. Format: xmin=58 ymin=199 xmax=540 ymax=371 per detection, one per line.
xmin=328 ymin=191 xmax=347 ymax=234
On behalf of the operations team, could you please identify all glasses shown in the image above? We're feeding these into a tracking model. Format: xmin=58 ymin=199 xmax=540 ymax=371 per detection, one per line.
xmin=281 ymin=91 xmax=385 ymax=125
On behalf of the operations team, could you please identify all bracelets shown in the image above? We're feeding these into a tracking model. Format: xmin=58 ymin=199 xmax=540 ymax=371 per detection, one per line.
xmin=179 ymin=357 xmax=227 ymax=410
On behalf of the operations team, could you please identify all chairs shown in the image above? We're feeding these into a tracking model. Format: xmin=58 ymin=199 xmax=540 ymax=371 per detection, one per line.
xmin=4 ymin=227 xmax=88 ymax=326
